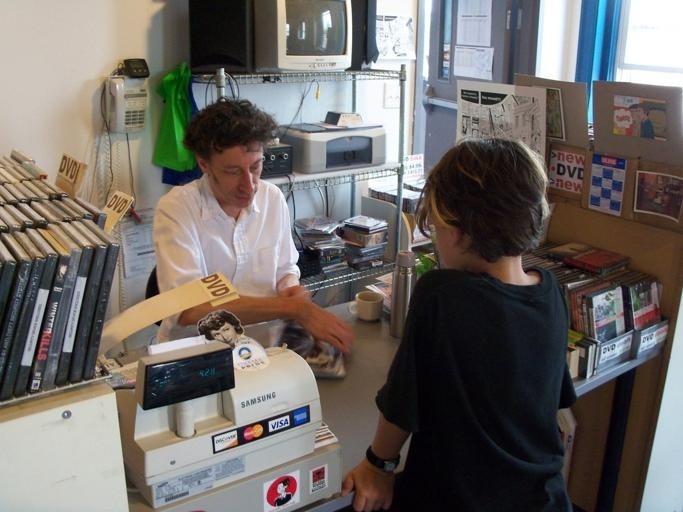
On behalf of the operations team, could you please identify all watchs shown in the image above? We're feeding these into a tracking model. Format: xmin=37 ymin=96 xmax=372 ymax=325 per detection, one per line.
xmin=364 ymin=445 xmax=403 ymax=472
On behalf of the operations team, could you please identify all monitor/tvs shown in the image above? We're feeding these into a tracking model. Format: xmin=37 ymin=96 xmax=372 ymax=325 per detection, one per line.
xmin=252 ymin=0 xmax=353 ymax=73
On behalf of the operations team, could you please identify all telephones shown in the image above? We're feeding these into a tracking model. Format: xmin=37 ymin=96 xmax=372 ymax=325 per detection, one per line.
xmin=105 ymin=75 xmax=150 ymax=134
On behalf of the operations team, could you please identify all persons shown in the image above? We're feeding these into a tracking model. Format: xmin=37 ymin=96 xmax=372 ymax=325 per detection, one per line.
xmin=151 ymin=97 xmax=353 ymax=357
xmin=636 ymin=102 xmax=655 ymax=140
xmin=196 ymin=309 xmax=261 ymax=350
xmin=629 ymin=104 xmax=641 ymax=136
xmin=342 ymin=135 xmax=571 ymax=510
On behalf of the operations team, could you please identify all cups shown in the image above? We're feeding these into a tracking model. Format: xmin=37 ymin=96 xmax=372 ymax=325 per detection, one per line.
xmin=346 ymin=289 xmax=385 ymax=321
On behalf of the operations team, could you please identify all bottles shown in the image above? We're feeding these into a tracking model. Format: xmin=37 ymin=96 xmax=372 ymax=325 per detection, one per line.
xmin=388 ymin=250 xmax=416 ymax=339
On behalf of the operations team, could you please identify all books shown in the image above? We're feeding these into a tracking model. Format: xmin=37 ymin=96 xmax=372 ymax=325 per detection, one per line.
xmin=291 ymin=214 xmax=390 ymax=272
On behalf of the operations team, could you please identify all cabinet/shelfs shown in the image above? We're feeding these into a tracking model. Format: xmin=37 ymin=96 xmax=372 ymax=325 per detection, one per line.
xmin=192 ymin=66 xmax=407 ymax=296
